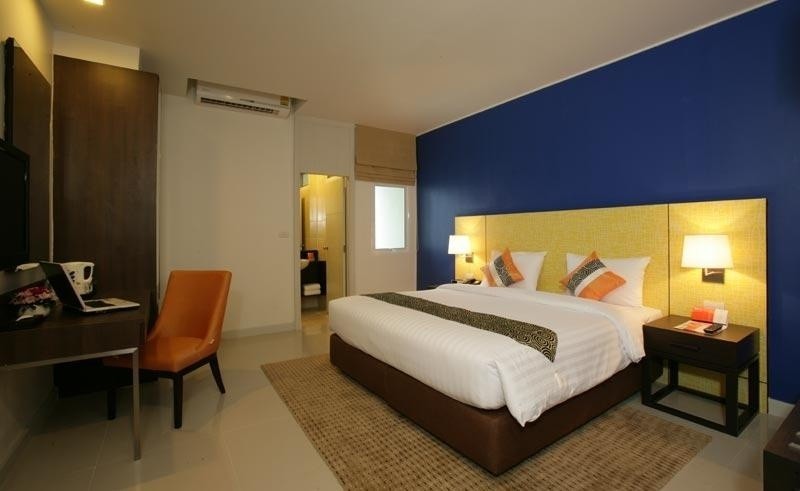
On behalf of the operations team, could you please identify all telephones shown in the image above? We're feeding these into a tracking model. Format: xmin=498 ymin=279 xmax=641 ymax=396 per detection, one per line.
xmin=462 ymin=279 xmax=481 ymax=285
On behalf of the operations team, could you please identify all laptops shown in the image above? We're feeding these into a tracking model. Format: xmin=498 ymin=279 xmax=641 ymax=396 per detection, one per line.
xmin=39 ymin=260 xmax=140 ymax=313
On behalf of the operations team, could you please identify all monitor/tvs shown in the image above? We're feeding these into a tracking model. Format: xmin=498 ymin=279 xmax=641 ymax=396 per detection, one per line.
xmin=0 ymin=137 xmax=31 ymax=266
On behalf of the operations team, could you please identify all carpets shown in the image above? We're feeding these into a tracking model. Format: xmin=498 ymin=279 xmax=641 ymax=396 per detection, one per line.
xmin=261 ymin=354 xmax=712 ymax=491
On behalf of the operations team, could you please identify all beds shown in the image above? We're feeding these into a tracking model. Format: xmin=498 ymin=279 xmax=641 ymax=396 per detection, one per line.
xmin=328 ymin=283 xmax=662 ymax=476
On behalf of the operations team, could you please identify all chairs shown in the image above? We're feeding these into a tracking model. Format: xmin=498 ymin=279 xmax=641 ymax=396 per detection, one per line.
xmin=103 ymin=270 xmax=232 ymax=429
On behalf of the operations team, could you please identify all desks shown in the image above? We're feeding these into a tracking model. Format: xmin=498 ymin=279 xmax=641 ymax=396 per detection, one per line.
xmin=763 ymin=398 xmax=800 ymax=491
xmin=0 ymin=298 xmax=142 ymax=461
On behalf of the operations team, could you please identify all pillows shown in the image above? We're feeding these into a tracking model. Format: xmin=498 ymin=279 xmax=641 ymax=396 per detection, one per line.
xmin=559 ymin=251 xmax=626 ymax=300
xmin=565 ymin=251 xmax=650 ymax=308
xmin=480 ymin=249 xmax=548 ymax=292
xmin=479 ymin=247 xmax=524 ymax=288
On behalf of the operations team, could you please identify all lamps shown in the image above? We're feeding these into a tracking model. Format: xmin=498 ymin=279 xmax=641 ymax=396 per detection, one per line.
xmin=681 ymin=235 xmax=734 ymax=277
xmin=447 ymin=234 xmax=471 ymax=257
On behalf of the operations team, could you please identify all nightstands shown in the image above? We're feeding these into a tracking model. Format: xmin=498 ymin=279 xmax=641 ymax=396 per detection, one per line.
xmin=641 ymin=315 xmax=760 ymax=438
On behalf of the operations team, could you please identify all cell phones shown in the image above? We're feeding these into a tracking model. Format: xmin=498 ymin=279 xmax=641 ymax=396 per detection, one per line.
xmin=704 ymin=323 xmax=722 ymax=333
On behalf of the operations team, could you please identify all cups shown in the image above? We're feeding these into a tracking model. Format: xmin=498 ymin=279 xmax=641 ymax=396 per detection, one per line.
xmin=308 ymin=253 xmax=314 ymax=260
